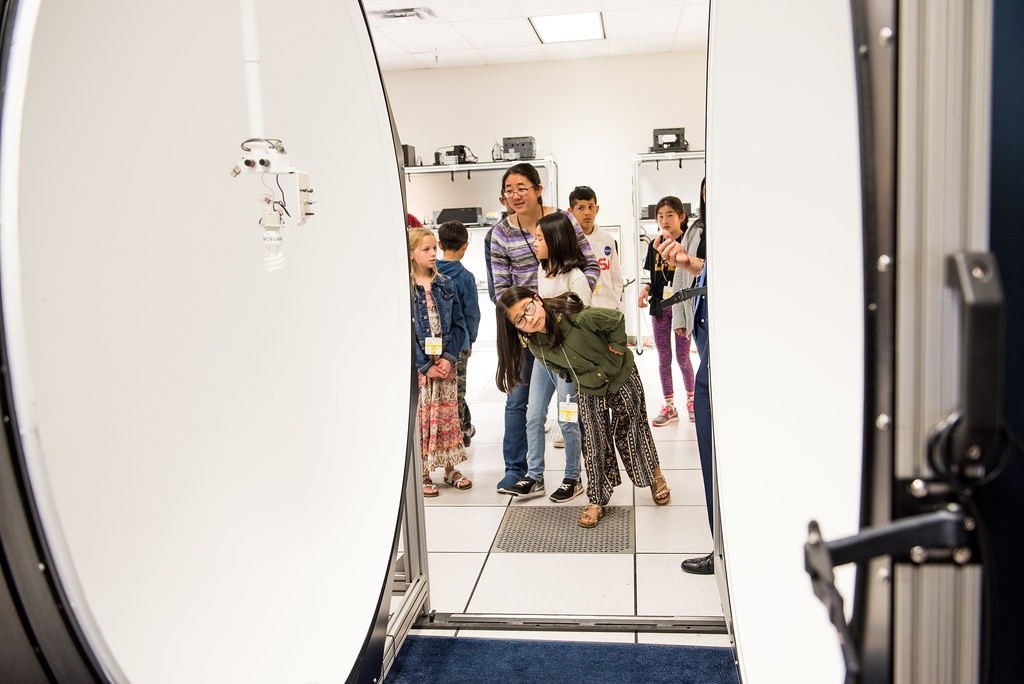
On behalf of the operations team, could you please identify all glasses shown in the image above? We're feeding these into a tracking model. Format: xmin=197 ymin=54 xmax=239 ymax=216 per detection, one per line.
xmin=514 ymin=298 xmax=536 ymax=329
xmin=502 ymin=185 xmax=534 ymax=199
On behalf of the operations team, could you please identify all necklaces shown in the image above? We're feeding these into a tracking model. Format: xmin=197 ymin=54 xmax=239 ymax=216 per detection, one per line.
xmin=536 ymin=331 xmax=580 ymax=423
xmin=661 ymin=236 xmax=672 ymax=300
xmin=517 ymin=204 xmax=543 ymax=265
xmin=425 ymin=331 xmax=442 ymax=356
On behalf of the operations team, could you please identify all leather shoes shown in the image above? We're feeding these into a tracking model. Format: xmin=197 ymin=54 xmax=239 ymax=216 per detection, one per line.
xmin=681 ymin=554 xmax=714 ymax=574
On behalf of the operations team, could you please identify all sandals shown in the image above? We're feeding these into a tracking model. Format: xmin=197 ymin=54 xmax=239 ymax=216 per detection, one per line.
xmin=444 ymin=470 xmax=472 ymax=490
xmin=577 ymin=502 xmax=606 ymax=528
xmin=650 ymin=472 xmax=670 ymax=505
xmin=421 ymin=476 xmax=439 ymax=498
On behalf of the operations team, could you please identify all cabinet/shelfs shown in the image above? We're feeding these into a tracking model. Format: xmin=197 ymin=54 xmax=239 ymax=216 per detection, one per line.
xmin=633 ymin=150 xmax=706 ymax=355
xmin=402 ymin=160 xmax=558 ymax=293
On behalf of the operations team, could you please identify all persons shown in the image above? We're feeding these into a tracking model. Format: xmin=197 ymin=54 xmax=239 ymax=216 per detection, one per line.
xmin=638 ymin=196 xmax=695 ymax=427
xmin=485 ymin=163 xmax=601 ymax=494
xmin=407 ymin=228 xmax=472 ymax=498
xmin=671 ymin=177 xmax=706 ymax=340
xmin=438 ymin=220 xmax=481 ymax=447
xmin=484 ymin=190 xmax=515 ymax=305
xmin=554 ymin=186 xmax=623 ymax=449
xmin=505 ymin=213 xmax=593 ymax=503
xmin=495 ymin=287 xmax=670 ymax=528
xmin=653 ymin=228 xmax=714 ymax=576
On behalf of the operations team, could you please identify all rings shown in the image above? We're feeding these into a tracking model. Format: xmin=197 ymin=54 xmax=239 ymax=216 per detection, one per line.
xmin=662 ymin=257 xmax=670 ymax=261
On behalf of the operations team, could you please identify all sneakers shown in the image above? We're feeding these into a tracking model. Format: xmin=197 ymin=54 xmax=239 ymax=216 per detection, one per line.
xmin=550 ymin=478 xmax=584 ymax=503
xmin=652 ymin=406 xmax=679 ymax=426
xmin=507 ymin=476 xmax=545 ymax=497
xmin=686 ymin=403 xmax=695 ymax=422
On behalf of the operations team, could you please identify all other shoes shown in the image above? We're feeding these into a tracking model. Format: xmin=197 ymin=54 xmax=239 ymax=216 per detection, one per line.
xmin=554 ymin=434 xmax=565 ymax=449
xmin=461 ymin=425 xmax=476 ymax=447
xmin=497 ymin=475 xmax=519 ymax=493
